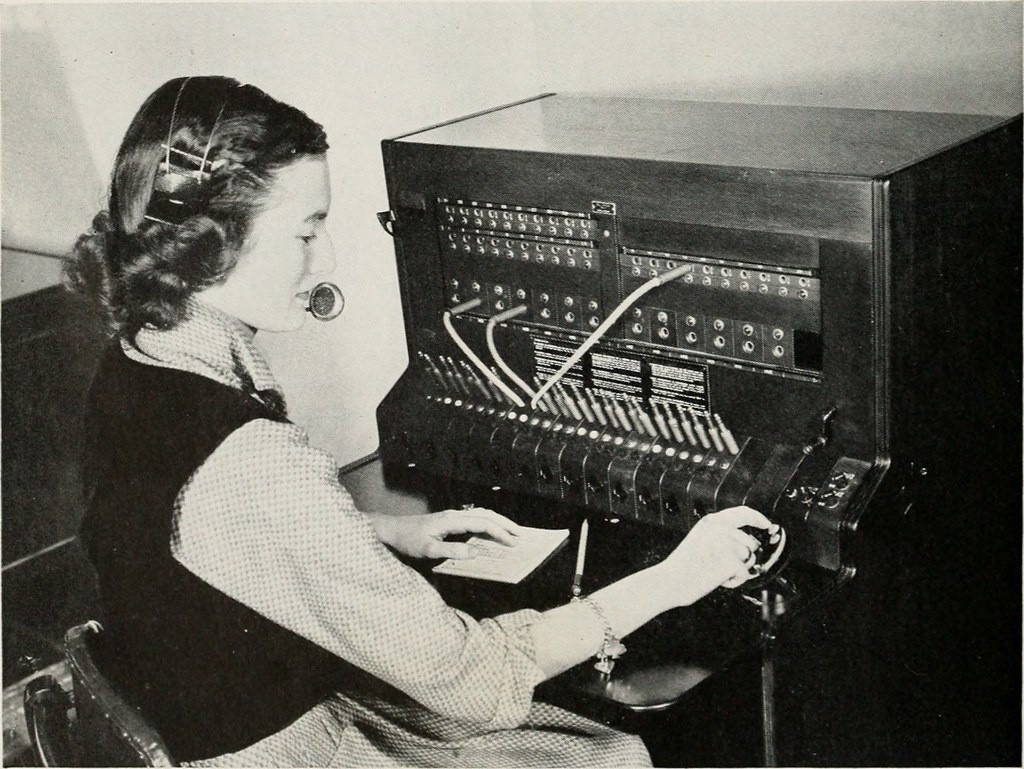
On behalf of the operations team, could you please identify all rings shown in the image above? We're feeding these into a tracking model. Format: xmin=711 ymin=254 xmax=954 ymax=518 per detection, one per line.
xmin=462 ymin=503 xmax=475 ymax=512
xmin=743 ymin=545 xmax=752 ymax=564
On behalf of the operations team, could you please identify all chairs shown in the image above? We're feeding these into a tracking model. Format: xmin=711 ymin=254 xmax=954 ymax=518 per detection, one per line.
xmin=24 ymin=620 xmax=180 ymax=767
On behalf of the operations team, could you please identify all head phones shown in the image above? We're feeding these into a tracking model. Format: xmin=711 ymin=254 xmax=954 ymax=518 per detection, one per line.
xmin=145 ymin=78 xmax=241 ymax=228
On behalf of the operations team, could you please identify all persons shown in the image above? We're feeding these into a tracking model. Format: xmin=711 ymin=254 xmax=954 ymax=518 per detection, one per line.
xmin=60 ymin=75 xmax=772 ymax=769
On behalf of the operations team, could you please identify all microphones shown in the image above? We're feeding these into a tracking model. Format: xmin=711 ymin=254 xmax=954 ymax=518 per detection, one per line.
xmin=304 ymin=282 xmax=344 ymax=322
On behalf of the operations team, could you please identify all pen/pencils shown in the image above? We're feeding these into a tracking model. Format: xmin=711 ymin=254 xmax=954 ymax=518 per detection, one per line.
xmin=569 ymin=517 xmax=589 ymax=600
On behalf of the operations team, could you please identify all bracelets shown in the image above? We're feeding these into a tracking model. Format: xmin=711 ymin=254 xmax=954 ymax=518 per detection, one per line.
xmin=570 ymin=595 xmax=627 ymax=682
